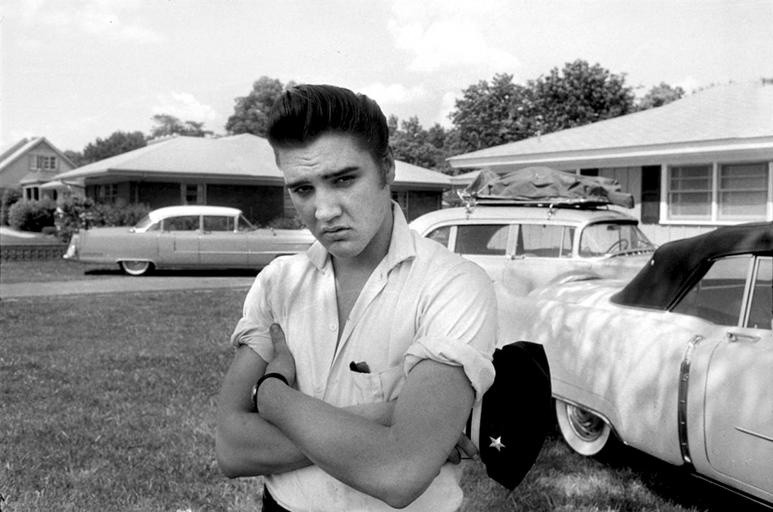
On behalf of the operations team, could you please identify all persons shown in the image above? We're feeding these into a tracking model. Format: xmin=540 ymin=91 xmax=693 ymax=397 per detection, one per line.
xmin=214 ymin=84 xmax=499 ymax=512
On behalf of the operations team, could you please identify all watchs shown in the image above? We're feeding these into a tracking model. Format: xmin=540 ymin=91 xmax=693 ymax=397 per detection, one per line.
xmin=249 ymin=373 xmax=289 ymax=413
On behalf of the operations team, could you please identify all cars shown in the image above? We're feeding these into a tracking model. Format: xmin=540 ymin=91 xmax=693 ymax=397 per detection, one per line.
xmin=62 ymin=204 xmax=316 ymax=276
xmin=519 ymin=223 xmax=771 ymax=510
xmin=406 ymin=164 xmax=657 ymax=349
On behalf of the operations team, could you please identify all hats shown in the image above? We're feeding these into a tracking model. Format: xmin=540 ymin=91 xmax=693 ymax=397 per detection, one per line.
xmin=456 ymin=341 xmax=552 ymax=491
